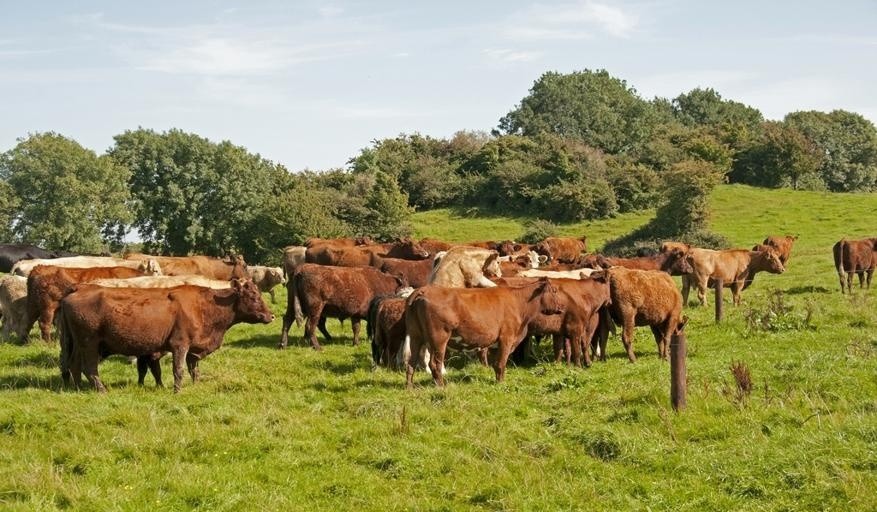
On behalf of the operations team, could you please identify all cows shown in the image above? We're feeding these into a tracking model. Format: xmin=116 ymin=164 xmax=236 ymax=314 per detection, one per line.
xmin=832 ymin=237 xmax=877 ymax=295
xmin=752 ymin=242 xmax=791 ymax=259
xmin=0 ymin=244 xmax=287 ymax=394
xmin=277 ymin=235 xmax=694 ymax=390
xmin=681 ymin=248 xmax=785 ymax=308
xmin=753 ymin=235 xmax=798 ymax=268
